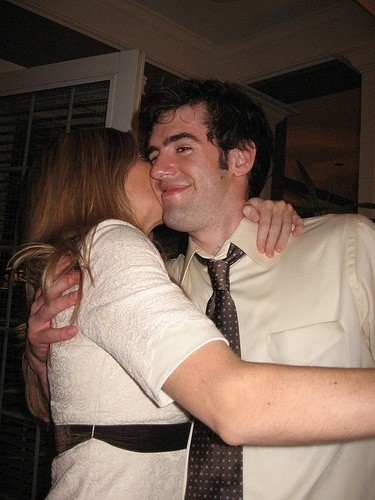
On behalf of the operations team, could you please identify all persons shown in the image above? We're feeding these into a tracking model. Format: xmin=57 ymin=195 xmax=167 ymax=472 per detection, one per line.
xmin=22 ymin=72 xmax=375 ymax=500
xmin=19 ymin=124 xmax=375 ymax=500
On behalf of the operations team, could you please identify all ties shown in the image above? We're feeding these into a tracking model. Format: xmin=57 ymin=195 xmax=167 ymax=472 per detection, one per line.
xmin=185 ymin=243 xmax=247 ymax=498
